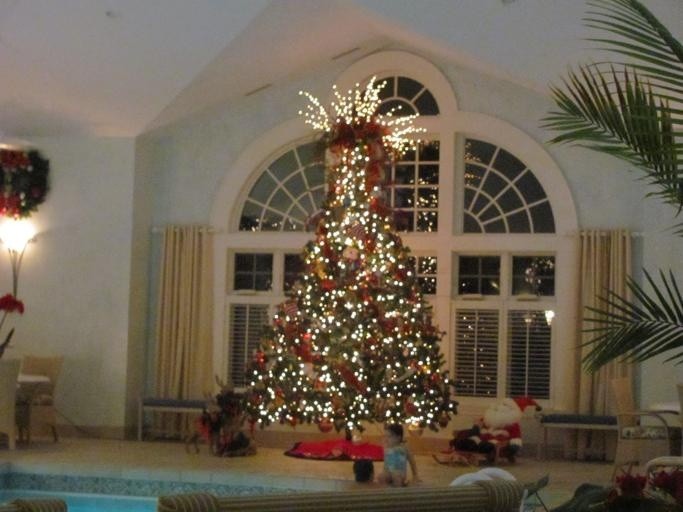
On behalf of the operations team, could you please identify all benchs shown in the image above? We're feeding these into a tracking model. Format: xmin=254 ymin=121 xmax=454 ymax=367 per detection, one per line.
xmin=540 ymin=414 xmax=617 ymax=463
xmin=136 ymin=397 xmax=224 ymax=441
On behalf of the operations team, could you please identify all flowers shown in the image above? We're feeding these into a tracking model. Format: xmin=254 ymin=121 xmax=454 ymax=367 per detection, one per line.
xmin=0 ymin=149 xmax=47 ymax=221
xmin=0 ymin=293 xmax=24 ymax=327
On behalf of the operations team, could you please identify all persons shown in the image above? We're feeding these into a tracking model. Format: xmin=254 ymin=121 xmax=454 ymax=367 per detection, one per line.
xmin=342 ymin=460 xmax=383 ymax=492
xmin=450 ymin=395 xmax=542 ymax=458
xmin=368 ymin=423 xmax=424 ymax=489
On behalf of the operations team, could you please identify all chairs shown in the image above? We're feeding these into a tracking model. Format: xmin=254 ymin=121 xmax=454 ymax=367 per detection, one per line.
xmin=452 ymin=428 xmax=517 ymax=467
xmin=0 ymin=353 xmax=59 ymax=450
xmin=609 ymin=376 xmax=681 ymax=489
